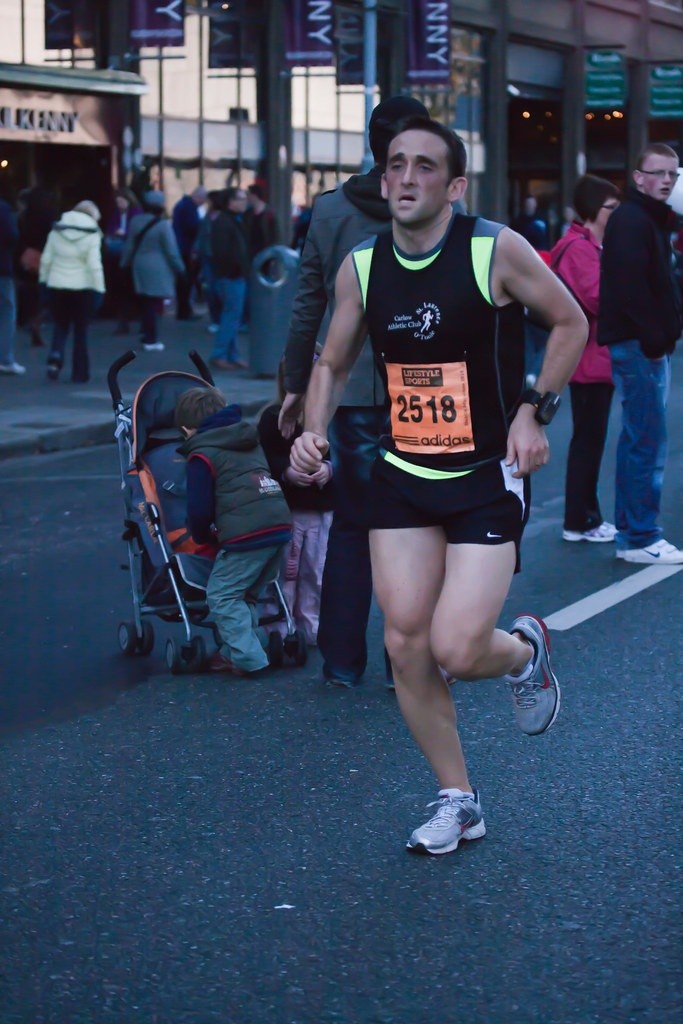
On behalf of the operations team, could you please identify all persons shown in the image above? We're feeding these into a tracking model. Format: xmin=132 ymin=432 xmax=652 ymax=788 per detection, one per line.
xmin=288 ymin=116 xmax=591 ymax=856
xmin=279 ymin=97 xmax=467 ymax=690
xmin=597 ymin=144 xmax=682 ymax=565
xmin=513 ymin=197 xmax=579 ymax=257
xmin=173 ymin=387 xmax=293 ymax=676
xmin=551 ymin=176 xmax=619 ymax=543
xmin=0 ymin=183 xmax=317 ymax=382
xmin=258 ymin=340 xmax=335 ymax=648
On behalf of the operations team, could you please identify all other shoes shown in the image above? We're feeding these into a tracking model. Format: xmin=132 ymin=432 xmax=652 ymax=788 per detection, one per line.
xmin=231 ymin=361 xmax=248 ymax=370
xmin=322 ymin=674 xmax=355 ymax=691
xmin=0 ymin=363 xmax=27 ymax=376
xmin=47 ymin=360 xmax=60 ymax=380
xmin=73 ymin=368 xmax=90 ymax=383
xmin=208 ymin=650 xmax=251 ymax=677
xmin=383 ymin=654 xmax=450 ymax=690
xmin=143 ymin=343 xmax=164 ymax=352
xmin=211 ymin=359 xmax=235 ymax=371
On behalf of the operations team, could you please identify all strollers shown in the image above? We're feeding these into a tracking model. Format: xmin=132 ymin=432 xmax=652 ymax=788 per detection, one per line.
xmin=108 ymin=349 xmax=306 ymax=675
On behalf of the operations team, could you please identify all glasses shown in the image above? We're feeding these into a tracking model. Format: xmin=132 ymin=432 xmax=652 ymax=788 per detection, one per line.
xmin=600 ymin=203 xmax=619 ymax=211
xmin=634 ymin=167 xmax=680 ymax=181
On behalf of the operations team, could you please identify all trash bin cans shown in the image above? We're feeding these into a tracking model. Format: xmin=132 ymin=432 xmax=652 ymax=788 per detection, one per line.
xmin=247 ymin=245 xmax=300 ymax=378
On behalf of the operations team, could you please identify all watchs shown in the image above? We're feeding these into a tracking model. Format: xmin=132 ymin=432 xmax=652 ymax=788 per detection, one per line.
xmin=520 ymin=387 xmax=562 ymax=424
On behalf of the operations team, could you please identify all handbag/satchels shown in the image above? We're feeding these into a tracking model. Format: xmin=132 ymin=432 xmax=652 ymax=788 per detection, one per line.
xmin=524 ymin=238 xmax=602 ymax=336
xmin=20 ymin=247 xmax=43 ymax=274
xmin=104 ymin=234 xmax=125 ymax=252
xmin=119 ymin=263 xmax=135 ymax=298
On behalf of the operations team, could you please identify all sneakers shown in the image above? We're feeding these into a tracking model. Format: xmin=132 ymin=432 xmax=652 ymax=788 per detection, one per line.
xmin=563 ymin=521 xmax=619 ymax=543
xmin=616 ymin=539 xmax=683 ymax=565
xmin=406 ymin=788 xmax=487 ymax=856
xmin=503 ymin=613 xmax=561 ymax=735
